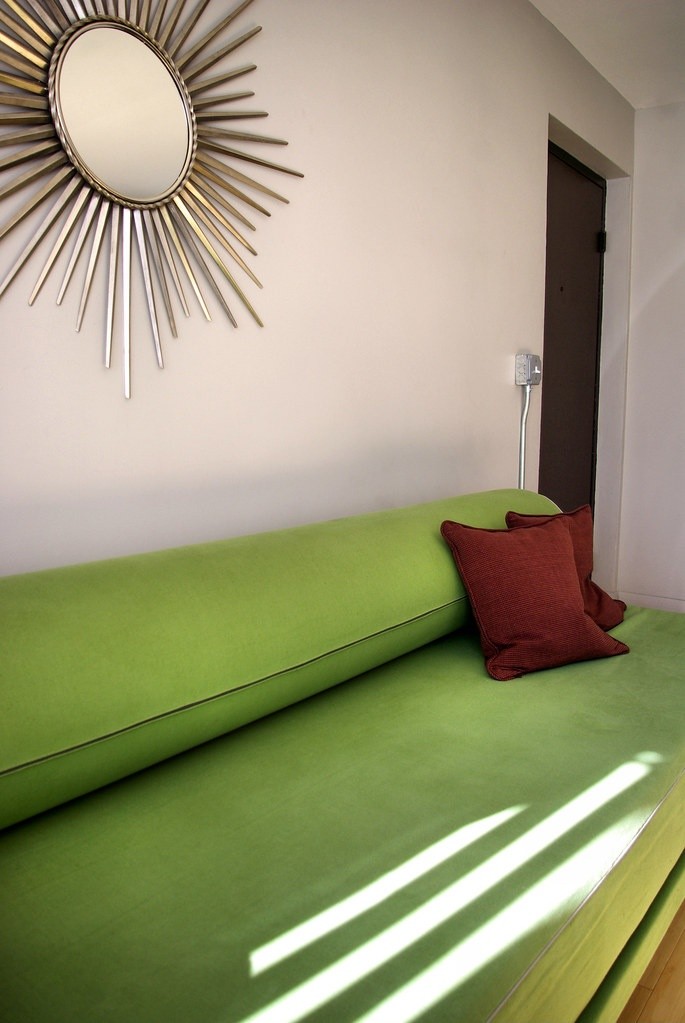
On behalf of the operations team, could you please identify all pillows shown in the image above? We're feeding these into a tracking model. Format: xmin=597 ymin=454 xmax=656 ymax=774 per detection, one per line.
xmin=505 ymin=503 xmax=628 ymax=632
xmin=438 ymin=516 xmax=630 ymax=681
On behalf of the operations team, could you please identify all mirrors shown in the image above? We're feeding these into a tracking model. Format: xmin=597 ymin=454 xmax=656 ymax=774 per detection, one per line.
xmin=0 ymin=0 xmax=304 ymax=399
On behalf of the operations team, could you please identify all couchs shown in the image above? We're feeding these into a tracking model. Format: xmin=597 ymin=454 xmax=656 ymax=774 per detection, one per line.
xmin=0 ymin=488 xmax=685 ymax=1023
